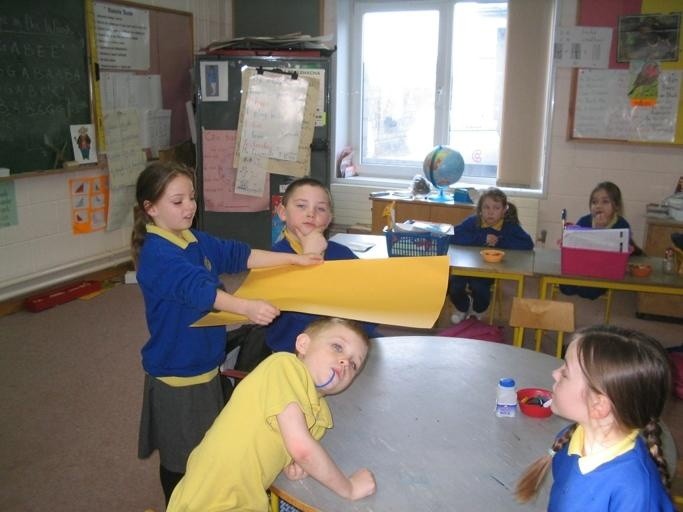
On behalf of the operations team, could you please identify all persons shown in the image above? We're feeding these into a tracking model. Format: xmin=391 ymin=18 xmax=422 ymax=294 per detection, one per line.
xmin=76 ymin=127 xmax=92 ymax=159
xmin=208 ymin=66 xmax=218 ymax=95
xmin=251 ymin=178 xmax=386 ymax=358
xmin=514 ymin=322 xmax=677 ymax=510
xmin=126 ymin=157 xmax=325 ymax=510
xmin=560 ymin=181 xmax=638 ymax=300
xmin=163 ymin=315 xmax=378 ymax=509
xmin=445 ymin=188 xmax=537 ymax=324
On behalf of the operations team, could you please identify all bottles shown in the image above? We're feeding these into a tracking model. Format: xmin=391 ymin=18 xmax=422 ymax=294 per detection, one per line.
xmin=662 ymin=248 xmax=676 ymax=274
xmin=495 ymin=378 xmax=518 ymax=418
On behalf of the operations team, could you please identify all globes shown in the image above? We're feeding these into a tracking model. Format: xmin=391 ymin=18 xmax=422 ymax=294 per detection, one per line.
xmin=423 ymin=146 xmax=464 ymax=201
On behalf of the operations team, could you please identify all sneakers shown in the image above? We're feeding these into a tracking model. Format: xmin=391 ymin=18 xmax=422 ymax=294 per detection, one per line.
xmin=451 ymin=294 xmax=486 ymax=325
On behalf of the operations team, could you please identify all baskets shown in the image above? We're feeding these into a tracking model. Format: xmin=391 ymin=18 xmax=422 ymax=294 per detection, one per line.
xmin=382 ymin=222 xmax=451 ymax=257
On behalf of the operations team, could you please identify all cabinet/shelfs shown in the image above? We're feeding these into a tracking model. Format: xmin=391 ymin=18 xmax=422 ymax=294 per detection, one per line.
xmin=368 ymin=190 xmax=477 ymax=233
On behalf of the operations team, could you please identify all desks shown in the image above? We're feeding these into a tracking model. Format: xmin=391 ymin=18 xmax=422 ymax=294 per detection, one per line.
xmin=269 ymin=336 xmax=679 ymax=511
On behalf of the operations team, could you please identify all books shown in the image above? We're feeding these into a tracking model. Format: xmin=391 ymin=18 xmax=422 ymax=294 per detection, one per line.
xmin=391 ymin=219 xmax=454 ymax=256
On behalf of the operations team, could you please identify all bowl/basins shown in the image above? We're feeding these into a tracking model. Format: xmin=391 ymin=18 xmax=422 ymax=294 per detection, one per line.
xmin=630 ymin=263 xmax=651 ymax=277
xmin=515 ymin=389 xmax=556 ymax=417
xmin=479 ymin=250 xmax=505 ymax=263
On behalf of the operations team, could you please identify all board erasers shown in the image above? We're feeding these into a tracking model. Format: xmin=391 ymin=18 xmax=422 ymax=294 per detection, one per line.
xmin=62 ymin=161 xmax=80 ymax=170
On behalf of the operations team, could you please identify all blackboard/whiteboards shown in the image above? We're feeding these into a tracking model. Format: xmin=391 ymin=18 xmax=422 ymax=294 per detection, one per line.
xmin=0 ymin=0 xmax=107 ymax=181
